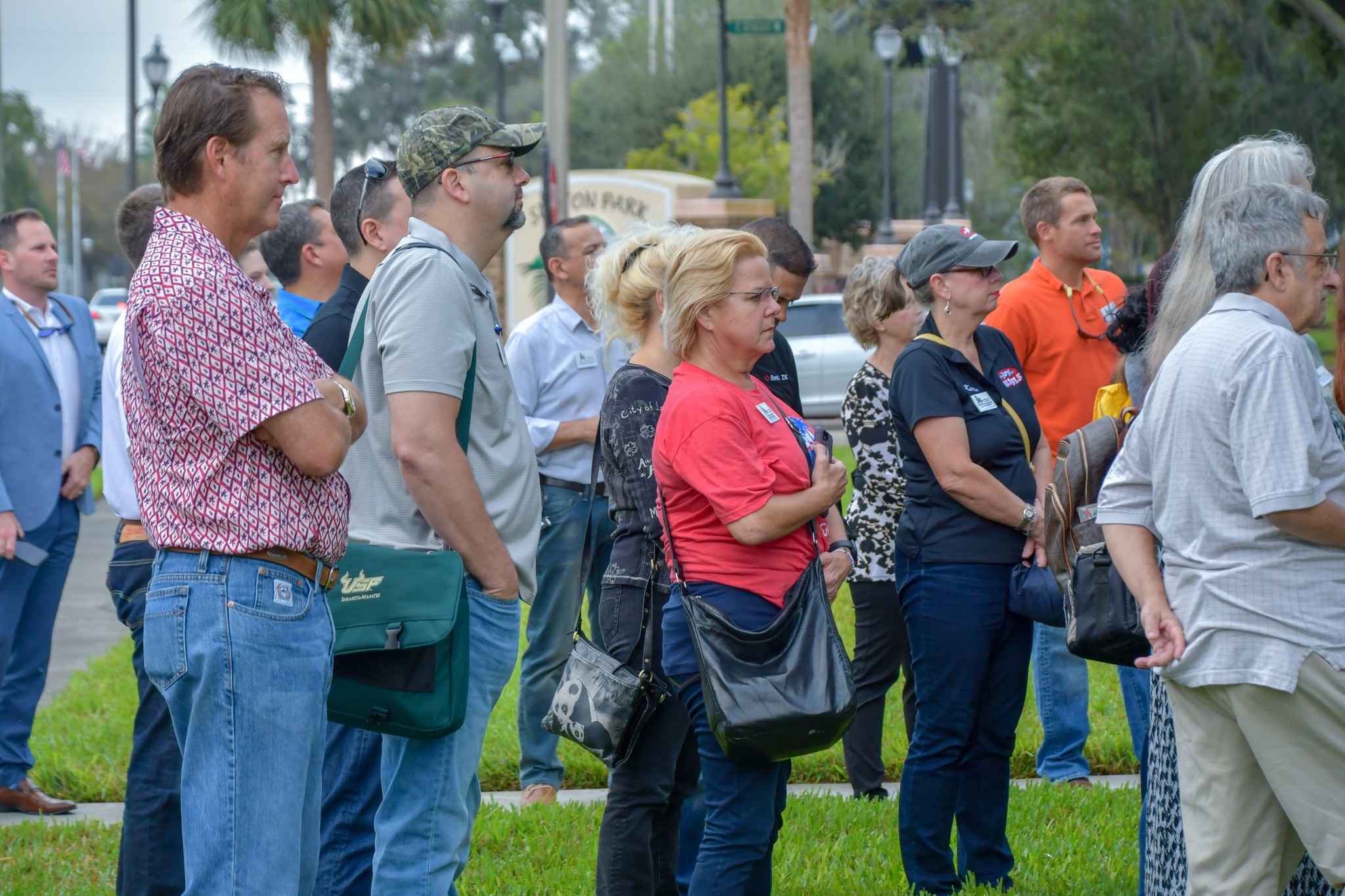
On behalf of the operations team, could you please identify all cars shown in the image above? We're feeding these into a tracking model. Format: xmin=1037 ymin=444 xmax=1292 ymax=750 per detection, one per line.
xmin=777 ymin=293 xmax=985 ymax=417
xmin=88 ymin=288 xmax=130 ymax=348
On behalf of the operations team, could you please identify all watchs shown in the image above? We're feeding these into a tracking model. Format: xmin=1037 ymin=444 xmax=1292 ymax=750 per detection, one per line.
xmin=1013 ymin=502 xmax=1033 ymax=532
xmin=828 ymin=539 xmax=858 ymax=567
xmin=332 ymin=379 xmax=356 ymax=420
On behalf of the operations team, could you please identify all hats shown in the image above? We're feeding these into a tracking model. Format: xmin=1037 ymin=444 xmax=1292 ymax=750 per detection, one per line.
xmin=896 ymin=219 xmax=1022 ymax=289
xmin=395 ymin=105 xmax=549 ymax=199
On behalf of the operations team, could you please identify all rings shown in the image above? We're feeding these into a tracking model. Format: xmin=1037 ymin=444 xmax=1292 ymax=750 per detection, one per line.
xmin=79 ymin=490 xmax=83 ymax=495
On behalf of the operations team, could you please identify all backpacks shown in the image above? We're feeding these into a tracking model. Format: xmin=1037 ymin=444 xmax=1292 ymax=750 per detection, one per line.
xmin=1038 ymin=398 xmax=1150 ymax=668
xmin=1085 ymin=376 xmax=1136 ymax=439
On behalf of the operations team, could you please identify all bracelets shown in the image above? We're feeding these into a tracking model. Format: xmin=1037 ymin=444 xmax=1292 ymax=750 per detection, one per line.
xmin=832 ymin=547 xmax=854 ymax=574
xmin=1023 ymin=504 xmax=1035 ymax=536
xmin=89 ymin=445 xmax=99 ymax=460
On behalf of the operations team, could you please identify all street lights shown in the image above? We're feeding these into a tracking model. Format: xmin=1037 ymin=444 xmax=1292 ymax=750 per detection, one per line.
xmin=874 ymin=20 xmax=965 ymax=242
xmin=126 ymin=0 xmax=172 ymax=195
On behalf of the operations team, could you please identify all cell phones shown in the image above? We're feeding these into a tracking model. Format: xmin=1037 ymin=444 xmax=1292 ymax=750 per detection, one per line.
xmin=813 ymin=426 xmax=833 ymax=472
xmin=13 ymin=535 xmax=48 ymax=567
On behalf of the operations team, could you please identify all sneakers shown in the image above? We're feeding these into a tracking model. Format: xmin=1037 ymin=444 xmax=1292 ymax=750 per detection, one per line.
xmin=1059 ymin=770 xmax=1096 ymax=796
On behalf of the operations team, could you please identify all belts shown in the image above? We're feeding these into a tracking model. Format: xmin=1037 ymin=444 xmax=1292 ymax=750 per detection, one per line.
xmin=537 ymin=471 xmax=613 ymax=501
xmin=116 ymin=511 xmax=146 ymax=535
xmin=164 ymin=534 xmax=338 ymax=599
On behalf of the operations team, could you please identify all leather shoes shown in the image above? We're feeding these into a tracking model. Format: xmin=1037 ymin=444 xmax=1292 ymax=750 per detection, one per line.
xmin=1 ymin=778 xmax=78 ymax=817
xmin=517 ymin=782 xmax=565 ymax=819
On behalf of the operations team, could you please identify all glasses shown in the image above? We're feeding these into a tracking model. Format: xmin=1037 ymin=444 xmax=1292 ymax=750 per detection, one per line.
xmin=1264 ymin=246 xmax=1339 ymax=284
xmin=435 ymin=149 xmax=518 ymax=191
xmin=1067 ymin=282 xmax=1121 ymax=348
xmin=355 ymin=157 xmax=389 ymax=249
xmin=717 ymin=282 xmax=780 ymax=308
xmin=9 ymin=289 xmax=78 ymax=338
xmin=931 ymin=257 xmax=994 ymax=278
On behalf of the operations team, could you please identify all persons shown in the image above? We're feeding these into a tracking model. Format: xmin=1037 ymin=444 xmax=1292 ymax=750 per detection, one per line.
xmin=261 ymin=198 xmax=350 ymax=339
xmin=233 ymin=238 xmax=274 ymax=297
xmin=1095 ymin=187 xmax=1345 ymax=896
xmin=650 ymin=229 xmax=857 ymax=895
xmin=344 ymin=104 xmax=540 ymax=895
xmin=581 ymin=223 xmax=705 ymax=895
xmin=301 ymin=159 xmax=414 ymax=895
xmin=102 ymin=181 xmax=169 ymax=895
xmin=124 ymin=63 xmax=367 ymax=895
xmin=503 ymin=217 xmax=635 ymax=808
xmin=842 ymin=256 xmax=928 ymax=798
xmin=675 ymin=218 xmax=817 ymax=895
xmin=0 ymin=208 xmax=103 ymax=816
xmin=1108 ymin=251 xmax=1179 ymax=896
xmin=890 ymin=225 xmax=1050 ymax=896
xmin=986 ymin=177 xmax=1151 ymax=788
xmin=1142 ymin=135 xmax=1345 ymax=896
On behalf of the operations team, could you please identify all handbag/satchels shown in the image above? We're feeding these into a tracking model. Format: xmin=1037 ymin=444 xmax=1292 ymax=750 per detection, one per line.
xmin=1008 ymin=551 xmax=1068 ymax=628
xmin=1059 ymin=541 xmax=1169 ymax=667
xmin=301 ymin=525 xmax=474 ymax=742
xmin=538 ymin=632 xmax=668 ymax=773
xmin=673 ymin=557 xmax=857 ymax=764
